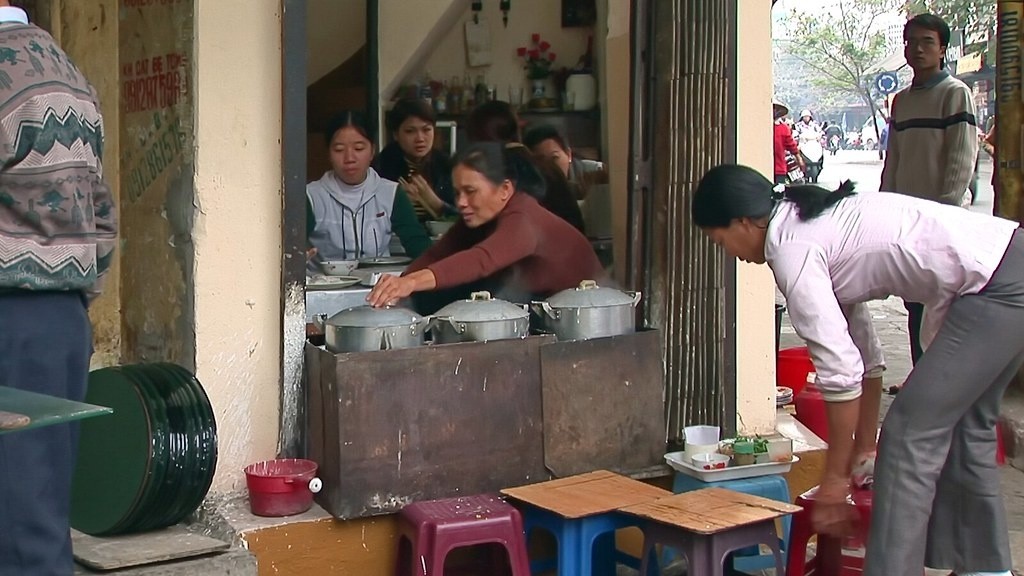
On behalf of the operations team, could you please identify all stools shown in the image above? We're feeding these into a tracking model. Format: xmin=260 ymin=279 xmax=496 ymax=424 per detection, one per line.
xmin=640 ymin=517 xmax=784 ymax=576
xmin=661 ymin=471 xmax=795 ymax=571
xmin=786 ymin=478 xmax=927 ymax=576
xmin=522 ymin=502 xmax=659 ymax=576
xmin=395 ymin=493 xmax=529 ymax=576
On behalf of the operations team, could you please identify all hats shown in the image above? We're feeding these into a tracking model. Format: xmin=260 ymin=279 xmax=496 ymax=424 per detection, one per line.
xmin=773 ymin=98 xmax=789 ymax=117
xmin=801 ymin=109 xmax=811 ymax=117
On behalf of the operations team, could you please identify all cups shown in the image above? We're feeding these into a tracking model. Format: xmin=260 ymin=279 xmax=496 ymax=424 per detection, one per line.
xmin=733 ymin=452 xmax=754 ymax=466
xmin=508 ymin=86 xmax=524 ymax=110
xmin=561 ymin=91 xmax=575 ymax=111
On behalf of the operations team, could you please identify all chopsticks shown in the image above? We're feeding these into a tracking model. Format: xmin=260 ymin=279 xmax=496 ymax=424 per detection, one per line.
xmin=398 ymin=176 xmax=438 ymax=218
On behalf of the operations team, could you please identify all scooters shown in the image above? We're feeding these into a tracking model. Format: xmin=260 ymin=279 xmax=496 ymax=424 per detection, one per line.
xmin=785 ymin=133 xmax=842 ymax=188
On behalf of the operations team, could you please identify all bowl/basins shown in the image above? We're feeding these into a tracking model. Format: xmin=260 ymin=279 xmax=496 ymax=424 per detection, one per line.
xmin=244 ymin=459 xmax=322 ymax=516
xmin=684 ymin=441 xmax=719 ymax=463
xmin=318 ymin=260 xmax=359 ymax=277
xmin=691 ymin=452 xmax=730 ymax=470
xmin=426 ymin=220 xmax=454 ymax=236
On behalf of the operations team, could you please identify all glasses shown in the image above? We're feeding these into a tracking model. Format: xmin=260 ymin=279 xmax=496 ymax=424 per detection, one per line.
xmin=903 ymin=38 xmax=935 ymax=47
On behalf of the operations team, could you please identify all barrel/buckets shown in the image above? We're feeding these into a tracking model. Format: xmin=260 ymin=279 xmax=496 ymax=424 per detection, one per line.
xmin=777 ymin=345 xmax=830 ymax=444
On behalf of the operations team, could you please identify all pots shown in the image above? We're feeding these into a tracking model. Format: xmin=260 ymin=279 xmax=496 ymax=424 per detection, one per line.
xmin=428 ymin=291 xmax=530 ymax=343
xmin=530 ymin=280 xmax=641 ymax=341
xmin=312 ymin=304 xmax=438 ymax=353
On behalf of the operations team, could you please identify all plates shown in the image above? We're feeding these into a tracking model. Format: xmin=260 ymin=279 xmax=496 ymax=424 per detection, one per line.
xmin=306 ymin=276 xmax=362 ymax=290
xmin=358 ymin=257 xmax=411 ymax=264
xmin=525 ymin=105 xmax=560 ymax=113
xmin=777 ymin=386 xmax=794 ymax=405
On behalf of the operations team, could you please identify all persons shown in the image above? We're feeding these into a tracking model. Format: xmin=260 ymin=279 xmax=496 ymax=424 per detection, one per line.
xmin=878 ymin=13 xmax=979 ymax=396
xmin=0 ymin=0 xmax=116 ymax=576
xmin=305 ymin=95 xmax=614 ymax=341
xmin=772 ymin=98 xmax=996 ymax=218
xmin=693 ymin=164 xmax=1024 ymax=576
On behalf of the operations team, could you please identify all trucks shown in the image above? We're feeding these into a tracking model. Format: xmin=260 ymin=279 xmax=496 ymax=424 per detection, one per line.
xmin=861 ymin=115 xmax=887 ymax=149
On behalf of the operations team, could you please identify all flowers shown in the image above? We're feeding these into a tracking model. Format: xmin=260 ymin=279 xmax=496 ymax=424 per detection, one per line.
xmin=515 ymin=32 xmax=556 ymax=76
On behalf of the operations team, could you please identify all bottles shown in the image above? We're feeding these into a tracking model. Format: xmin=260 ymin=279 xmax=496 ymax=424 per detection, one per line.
xmin=567 ymin=70 xmax=597 ymax=110
xmin=416 ymin=70 xmax=496 ymax=114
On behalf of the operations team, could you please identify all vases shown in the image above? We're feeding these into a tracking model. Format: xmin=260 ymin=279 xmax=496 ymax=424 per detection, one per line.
xmin=528 ymin=74 xmax=547 ymax=97
